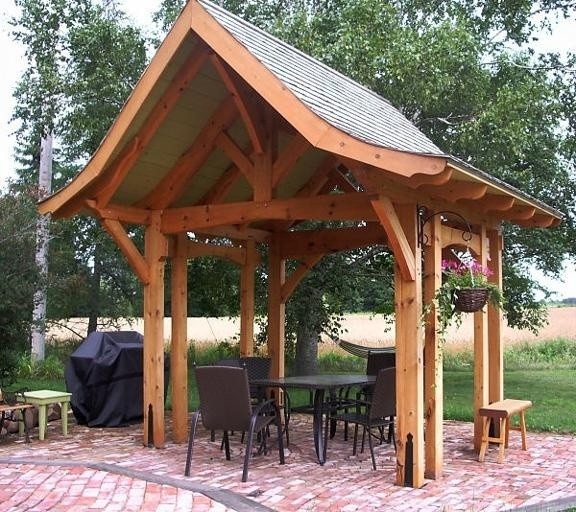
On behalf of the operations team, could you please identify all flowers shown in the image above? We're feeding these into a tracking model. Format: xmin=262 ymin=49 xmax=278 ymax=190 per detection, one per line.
xmin=441 ymin=258 xmax=495 ymax=281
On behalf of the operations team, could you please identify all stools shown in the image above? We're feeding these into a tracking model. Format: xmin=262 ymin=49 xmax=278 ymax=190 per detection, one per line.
xmin=15 ymin=388 xmax=72 ymax=440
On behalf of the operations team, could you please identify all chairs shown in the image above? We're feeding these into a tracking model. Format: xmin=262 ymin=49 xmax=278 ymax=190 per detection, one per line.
xmin=184 ymin=346 xmax=396 ymax=483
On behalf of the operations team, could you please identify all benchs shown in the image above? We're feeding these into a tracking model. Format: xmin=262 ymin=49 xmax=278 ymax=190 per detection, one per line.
xmin=476 ymin=398 xmax=534 ymax=465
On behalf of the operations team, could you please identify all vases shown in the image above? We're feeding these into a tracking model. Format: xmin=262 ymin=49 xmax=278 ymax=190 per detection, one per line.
xmin=449 ymin=286 xmax=490 ymax=313
